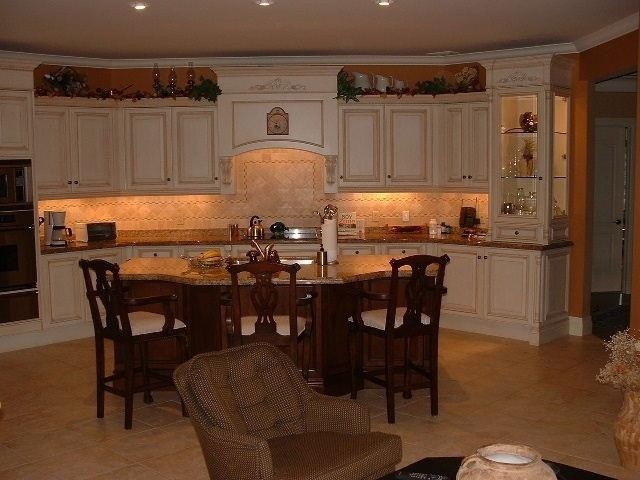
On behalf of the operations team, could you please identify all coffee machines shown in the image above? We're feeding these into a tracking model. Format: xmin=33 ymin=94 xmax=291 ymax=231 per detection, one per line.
xmin=43 ymin=211 xmax=71 ymax=247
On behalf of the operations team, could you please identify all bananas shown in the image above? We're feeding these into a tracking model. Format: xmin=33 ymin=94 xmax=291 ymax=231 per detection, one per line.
xmin=197 ymin=250 xmax=222 ymax=262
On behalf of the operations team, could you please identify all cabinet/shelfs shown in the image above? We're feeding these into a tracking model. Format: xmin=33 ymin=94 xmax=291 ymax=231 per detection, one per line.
xmin=174 ymin=106 xmax=221 ymax=196
xmin=487 ymin=57 xmax=576 ymax=245
xmin=440 ymin=101 xmax=492 ymax=193
xmin=337 ymin=242 xmax=425 ymax=256
xmin=122 ymin=108 xmax=171 ymax=195
xmin=437 ymin=247 xmax=571 ymax=349
xmin=42 ymin=247 xmax=225 ymax=345
xmin=385 ymin=101 xmax=433 ymax=193
xmin=27 ymin=104 xmax=118 ymax=202
xmin=0 ymin=88 xmax=34 ymax=162
xmin=338 ymin=101 xmax=386 ymax=194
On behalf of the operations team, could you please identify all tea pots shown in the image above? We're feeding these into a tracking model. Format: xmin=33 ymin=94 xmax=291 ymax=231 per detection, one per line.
xmin=246 ymin=214 xmax=264 ymax=238
xmin=373 ymin=74 xmax=395 ymax=92
xmin=393 ymin=79 xmax=409 ymax=89
xmin=350 ymin=71 xmax=375 ymax=91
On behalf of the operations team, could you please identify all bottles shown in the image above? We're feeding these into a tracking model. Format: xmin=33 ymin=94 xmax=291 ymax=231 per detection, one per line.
xmin=317 ymin=248 xmax=328 ymax=264
xmin=426 ymin=218 xmax=453 ymax=235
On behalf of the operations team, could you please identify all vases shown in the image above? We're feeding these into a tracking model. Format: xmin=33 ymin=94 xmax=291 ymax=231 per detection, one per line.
xmin=613 ymin=389 xmax=640 ymax=471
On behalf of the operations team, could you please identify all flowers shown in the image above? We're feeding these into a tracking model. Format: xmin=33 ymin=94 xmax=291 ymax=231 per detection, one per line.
xmin=594 ymin=326 xmax=640 ymax=393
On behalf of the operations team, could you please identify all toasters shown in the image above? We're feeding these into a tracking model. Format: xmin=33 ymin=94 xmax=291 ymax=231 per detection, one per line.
xmin=75 ymin=221 xmax=118 ymax=242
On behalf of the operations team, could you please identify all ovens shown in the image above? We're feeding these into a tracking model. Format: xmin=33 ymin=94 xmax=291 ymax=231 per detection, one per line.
xmin=0 ymin=160 xmax=42 ymax=325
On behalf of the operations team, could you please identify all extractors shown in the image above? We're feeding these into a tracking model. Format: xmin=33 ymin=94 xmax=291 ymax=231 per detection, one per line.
xmin=217 ymin=94 xmax=335 ymax=159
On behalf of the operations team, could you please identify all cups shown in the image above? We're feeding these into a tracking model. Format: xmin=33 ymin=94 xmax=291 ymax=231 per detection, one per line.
xmin=500 ymin=141 xmax=539 ymax=221
xmin=356 ymin=219 xmax=364 ymax=239
xmin=229 ymin=223 xmax=239 ymax=243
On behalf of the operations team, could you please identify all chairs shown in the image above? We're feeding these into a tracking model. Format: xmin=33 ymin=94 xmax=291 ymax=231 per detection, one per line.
xmin=78 ymin=259 xmax=191 ymax=430
xmin=172 ymin=343 xmax=403 ymax=480
xmin=217 ymin=261 xmax=318 ymax=384
xmin=344 ymin=255 xmax=450 ymax=424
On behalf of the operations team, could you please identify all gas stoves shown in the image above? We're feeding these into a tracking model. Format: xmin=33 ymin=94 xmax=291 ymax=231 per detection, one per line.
xmin=243 ymin=228 xmax=319 ymax=240
